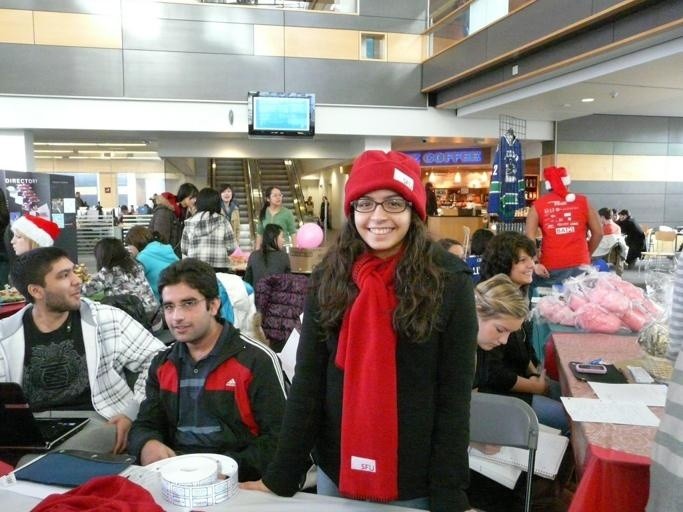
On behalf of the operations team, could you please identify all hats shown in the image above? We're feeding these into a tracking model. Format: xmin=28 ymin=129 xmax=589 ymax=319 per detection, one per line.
xmin=544 ymin=168 xmax=577 ymax=203
xmin=344 ymin=148 xmax=431 ymax=224
xmin=12 ymin=214 xmax=61 ymax=248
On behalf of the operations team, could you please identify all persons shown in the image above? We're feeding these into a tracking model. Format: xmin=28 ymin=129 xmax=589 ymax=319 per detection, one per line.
xmin=0 ymin=190 xmax=14 ymax=290
xmin=468 ymin=274 xmax=531 ymax=512
xmin=321 ymin=196 xmax=329 ymax=228
xmin=149 ymin=183 xmax=199 ymax=244
xmin=598 ymin=207 xmax=621 ymax=234
xmin=525 ymin=166 xmax=604 ymax=271
xmin=0 ymin=248 xmax=166 ymax=455
xmin=81 ymin=236 xmax=164 ymax=335
xmin=465 ymin=229 xmax=496 ymax=282
xmin=238 ymin=150 xmax=476 ymax=511
xmin=612 ymin=208 xmax=621 ymax=224
xmin=244 ymin=224 xmax=290 ymax=290
xmin=219 ymin=184 xmax=240 ymax=247
xmin=10 ymin=211 xmax=61 ymax=256
xmin=437 ymin=238 xmax=464 ymax=260
xmin=478 ymin=231 xmax=576 ymax=491
xmin=126 ymin=258 xmax=293 ymax=467
xmin=305 ymin=196 xmax=314 ymax=216
xmin=425 ymin=183 xmax=438 ymax=216
xmin=75 ymin=188 xmax=158 ymax=226
xmin=620 ymin=209 xmax=646 ymax=268
xmin=180 ymin=188 xmax=235 ymax=272
xmin=255 ymin=188 xmax=298 ymax=252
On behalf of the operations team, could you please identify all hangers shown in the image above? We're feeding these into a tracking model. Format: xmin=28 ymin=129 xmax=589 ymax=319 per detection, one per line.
xmin=504 ymin=128 xmax=516 ymax=139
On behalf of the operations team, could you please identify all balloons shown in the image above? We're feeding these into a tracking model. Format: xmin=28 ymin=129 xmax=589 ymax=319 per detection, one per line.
xmin=296 ymin=222 xmax=323 ymax=249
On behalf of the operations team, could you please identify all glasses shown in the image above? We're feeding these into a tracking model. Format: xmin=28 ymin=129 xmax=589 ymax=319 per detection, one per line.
xmin=270 ymin=193 xmax=283 ymax=198
xmin=159 ymin=298 xmax=208 ymax=311
xmin=349 ymin=198 xmax=413 ymax=213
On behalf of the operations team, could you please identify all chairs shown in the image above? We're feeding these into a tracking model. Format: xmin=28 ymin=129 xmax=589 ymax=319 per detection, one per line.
xmin=641 ymin=230 xmax=677 ymax=272
xmin=470 ymin=393 xmax=539 ymax=512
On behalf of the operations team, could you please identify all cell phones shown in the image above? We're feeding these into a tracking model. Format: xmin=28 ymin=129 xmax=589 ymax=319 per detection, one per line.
xmin=576 ymin=364 xmax=608 ymax=375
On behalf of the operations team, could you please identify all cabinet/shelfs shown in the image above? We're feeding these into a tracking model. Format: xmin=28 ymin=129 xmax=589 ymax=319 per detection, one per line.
xmin=488 ymin=174 xmax=539 ymax=234
xmin=432 ymin=186 xmax=490 ymax=215
xmin=428 ymin=215 xmax=484 ymax=243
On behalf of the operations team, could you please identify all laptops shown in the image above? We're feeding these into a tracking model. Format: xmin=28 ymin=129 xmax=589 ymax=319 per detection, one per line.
xmin=0 ymin=381 xmax=89 ymax=454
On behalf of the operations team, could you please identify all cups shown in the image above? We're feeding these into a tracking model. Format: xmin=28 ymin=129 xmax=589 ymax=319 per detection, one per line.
xmin=644 ymin=263 xmax=674 ymax=321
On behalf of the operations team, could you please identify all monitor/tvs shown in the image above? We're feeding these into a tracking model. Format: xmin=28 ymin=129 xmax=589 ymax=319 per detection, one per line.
xmin=247 ymin=91 xmax=316 ymax=137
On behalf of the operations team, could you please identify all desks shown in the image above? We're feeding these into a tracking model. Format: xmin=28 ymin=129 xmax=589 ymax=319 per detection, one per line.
xmin=0 ymin=288 xmax=29 ymax=319
xmin=532 ymin=285 xmax=675 ymax=511
xmin=1 ymin=452 xmax=429 ymax=512
